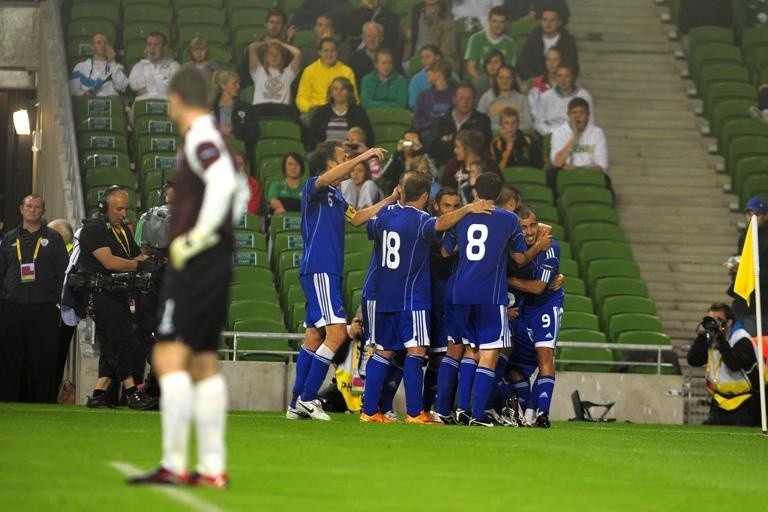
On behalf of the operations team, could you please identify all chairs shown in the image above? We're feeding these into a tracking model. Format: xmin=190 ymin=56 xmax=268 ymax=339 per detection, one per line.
xmin=658 ymin=1 xmax=768 ymax=215
xmin=61 ymin=0 xmax=310 ymax=361
xmin=282 ymin=0 xmax=682 ymax=376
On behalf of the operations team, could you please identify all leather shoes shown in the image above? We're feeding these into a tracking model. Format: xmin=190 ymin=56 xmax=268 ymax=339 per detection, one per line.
xmin=125 ymin=390 xmax=159 ymax=409
xmin=85 ymin=394 xmax=118 ymax=409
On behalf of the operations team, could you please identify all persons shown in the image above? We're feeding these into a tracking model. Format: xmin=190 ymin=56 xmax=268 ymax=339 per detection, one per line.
xmin=722 ymin=195 xmax=767 ymax=340
xmin=123 ymin=62 xmax=239 ymax=488
xmin=687 ymin=300 xmax=768 ymax=427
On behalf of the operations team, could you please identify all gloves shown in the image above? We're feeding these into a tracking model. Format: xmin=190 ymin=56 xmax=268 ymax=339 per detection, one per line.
xmin=168 ymin=232 xmax=220 ymax=271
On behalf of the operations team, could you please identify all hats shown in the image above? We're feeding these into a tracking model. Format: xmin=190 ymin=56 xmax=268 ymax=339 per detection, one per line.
xmin=743 ymin=195 xmax=768 ymax=215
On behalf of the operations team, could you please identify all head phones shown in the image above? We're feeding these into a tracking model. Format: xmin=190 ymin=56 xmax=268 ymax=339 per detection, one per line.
xmin=97 ymin=185 xmax=120 ymax=213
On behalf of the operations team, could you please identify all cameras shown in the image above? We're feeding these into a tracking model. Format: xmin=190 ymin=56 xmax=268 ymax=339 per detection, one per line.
xmin=345 ymin=144 xmax=360 ymax=150
xmin=356 ymin=319 xmax=367 ymax=341
xmin=402 ymin=140 xmax=413 ymax=147
xmin=701 ymin=316 xmax=726 ymax=332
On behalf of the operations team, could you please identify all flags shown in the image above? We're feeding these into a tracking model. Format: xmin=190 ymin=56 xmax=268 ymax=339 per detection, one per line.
xmin=733 ymin=219 xmax=758 ymax=308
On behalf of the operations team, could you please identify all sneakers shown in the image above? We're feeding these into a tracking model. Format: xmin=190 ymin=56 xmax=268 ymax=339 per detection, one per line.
xmin=435 ymin=411 xmax=459 ymax=425
xmin=497 ymin=406 xmax=551 ymax=428
xmin=188 ymin=467 xmax=228 ymax=488
xmin=383 ymin=410 xmax=400 ymax=422
xmin=125 ymin=463 xmax=191 ymax=487
xmin=455 ymin=407 xmax=495 ymax=427
xmin=295 ymin=395 xmax=331 ymax=421
xmin=404 ymin=409 xmax=442 ymax=424
xmin=359 ymin=411 xmax=392 ymax=423
xmin=285 ymin=403 xmax=310 ymax=420
